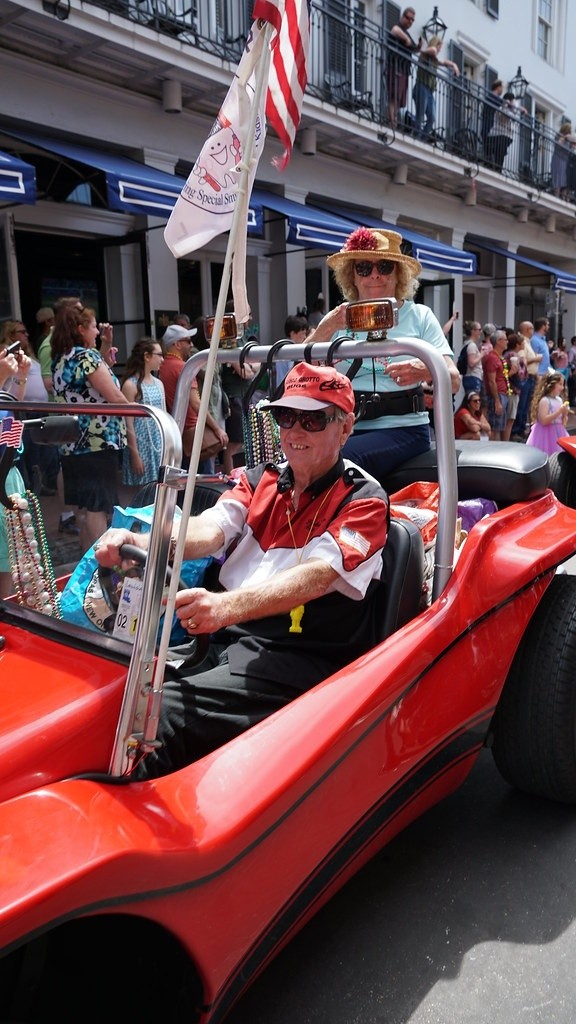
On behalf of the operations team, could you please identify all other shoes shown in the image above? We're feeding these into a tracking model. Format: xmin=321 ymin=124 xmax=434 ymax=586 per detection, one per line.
xmin=512 ymin=434 xmax=523 ymax=441
xmin=41 ymin=486 xmax=57 ymax=495
xmin=58 ymin=514 xmax=81 ymax=535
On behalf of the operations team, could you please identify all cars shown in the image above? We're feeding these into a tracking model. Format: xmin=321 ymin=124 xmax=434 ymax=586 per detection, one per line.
xmin=0 ymin=296 xmax=576 ymax=1020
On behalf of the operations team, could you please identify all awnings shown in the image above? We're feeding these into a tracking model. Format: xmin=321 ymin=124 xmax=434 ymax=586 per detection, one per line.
xmin=176 ymin=170 xmax=363 ymax=252
xmin=466 ymin=238 xmax=576 ymax=294
xmin=0 ymin=125 xmax=263 ymax=234
xmin=0 ymin=150 xmax=36 ymax=206
xmin=306 ymin=198 xmax=477 ymax=275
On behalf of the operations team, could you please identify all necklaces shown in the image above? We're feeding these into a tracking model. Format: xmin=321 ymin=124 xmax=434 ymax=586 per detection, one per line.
xmin=166 ymin=353 xmax=200 ymax=401
xmin=286 ymin=477 xmax=338 ymax=633
xmin=494 ymin=349 xmax=513 ymax=395
xmin=4 ymin=490 xmax=64 ymax=620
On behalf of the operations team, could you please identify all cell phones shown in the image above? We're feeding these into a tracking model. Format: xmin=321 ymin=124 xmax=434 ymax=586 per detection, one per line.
xmin=5 ymin=341 xmax=21 ymax=356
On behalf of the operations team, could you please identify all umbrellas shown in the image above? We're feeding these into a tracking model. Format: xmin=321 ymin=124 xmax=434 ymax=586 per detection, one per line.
xmin=239 ymin=337 xmax=363 ymax=470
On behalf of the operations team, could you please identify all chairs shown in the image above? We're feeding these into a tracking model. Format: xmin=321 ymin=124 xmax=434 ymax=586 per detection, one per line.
xmin=324 ymin=74 xmax=374 ymax=120
xmin=371 ymin=517 xmax=424 ymax=640
xmin=404 ymin=111 xmax=446 ymax=143
xmin=522 ymin=165 xmax=552 ymax=190
xmin=140 ymin=0 xmax=198 ymax=34
xmin=226 ymin=34 xmax=246 ymax=62
xmin=126 ymin=477 xmax=229 ymax=592
xmin=454 ymin=128 xmax=483 ymax=160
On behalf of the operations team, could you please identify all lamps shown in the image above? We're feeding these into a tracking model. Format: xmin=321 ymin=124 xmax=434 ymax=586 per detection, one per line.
xmin=517 ymin=209 xmax=528 ymax=223
xmin=507 ymin=66 xmax=529 ymax=100
xmin=422 ymin=5 xmax=447 ymax=44
xmin=545 ymin=216 xmax=556 ymax=233
xmin=465 ymin=190 xmax=477 ymax=206
xmin=392 ymin=161 xmax=409 ymax=185
xmin=162 ymin=79 xmax=183 ymax=114
xmin=301 ymin=129 xmax=317 ymax=155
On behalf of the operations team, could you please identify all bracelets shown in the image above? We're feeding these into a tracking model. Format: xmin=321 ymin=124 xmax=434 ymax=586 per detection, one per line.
xmin=12 ymin=377 xmax=27 ymax=384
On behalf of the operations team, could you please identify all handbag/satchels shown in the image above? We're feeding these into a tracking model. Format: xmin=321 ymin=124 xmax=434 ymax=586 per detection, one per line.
xmin=181 ymin=422 xmax=221 ymax=461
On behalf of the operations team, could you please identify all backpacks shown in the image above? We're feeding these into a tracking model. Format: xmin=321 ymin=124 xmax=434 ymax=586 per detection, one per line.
xmin=456 ymin=343 xmax=470 ymax=375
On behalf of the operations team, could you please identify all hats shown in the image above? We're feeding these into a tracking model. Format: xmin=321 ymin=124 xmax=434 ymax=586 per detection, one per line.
xmin=163 ymin=324 xmax=197 ymax=346
xmin=326 ymin=228 xmax=422 ymax=278
xmin=257 ymin=360 xmax=355 ymax=415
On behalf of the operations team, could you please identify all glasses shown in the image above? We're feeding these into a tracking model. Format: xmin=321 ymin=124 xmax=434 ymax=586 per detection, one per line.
xmin=173 ymin=339 xmax=191 ymax=346
xmin=13 ymin=329 xmax=27 ymax=335
xmin=146 ymin=352 xmax=165 ymax=358
xmin=271 ymin=407 xmax=344 ymax=432
xmin=353 ymin=259 xmax=396 ymax=277
xmin=39 ymin=316 xmax=56 ymax=327
xmin=470 ymin=399 xmax=481 ymax=403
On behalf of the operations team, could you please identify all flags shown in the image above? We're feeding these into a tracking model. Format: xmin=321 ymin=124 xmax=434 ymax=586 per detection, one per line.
xmin=164 ymin=0 xmax=313 ymax=258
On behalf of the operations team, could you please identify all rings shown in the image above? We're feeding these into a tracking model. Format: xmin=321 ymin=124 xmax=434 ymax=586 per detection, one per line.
xmin=188 ymin=614 xmax=202 ymax=629
xmin=93 ymin=544 xmax=101 ymax=551
xmin=397 ymin=377 xmax=401 ymax=383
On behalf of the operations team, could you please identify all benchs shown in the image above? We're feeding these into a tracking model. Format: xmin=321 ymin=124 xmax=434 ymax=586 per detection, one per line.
xmin=382 ymin=437 xmax=552 ymax=499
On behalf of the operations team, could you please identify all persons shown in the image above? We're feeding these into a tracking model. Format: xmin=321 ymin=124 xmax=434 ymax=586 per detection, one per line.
xmin=412 ymin=35 xmax=460 ymax=143
xmin=481 ymin=80 xmax=528 ymax=173
xmin=274 ymin=301 xmax=325 ymax=390
xmin=0 ymin=297 xmax=270 ymax=599
xmin=550 ymin=124 xmax=576 ymax=205
xmin=442 ymin=310 xmax=576 ymax=457
xmin=384 ymin=7 xmax=424 ymax=131
xmin=93 ymin=362 xmax=391 ymax=780
xmin=301 ymin=228 xmax=461 ymax=479
